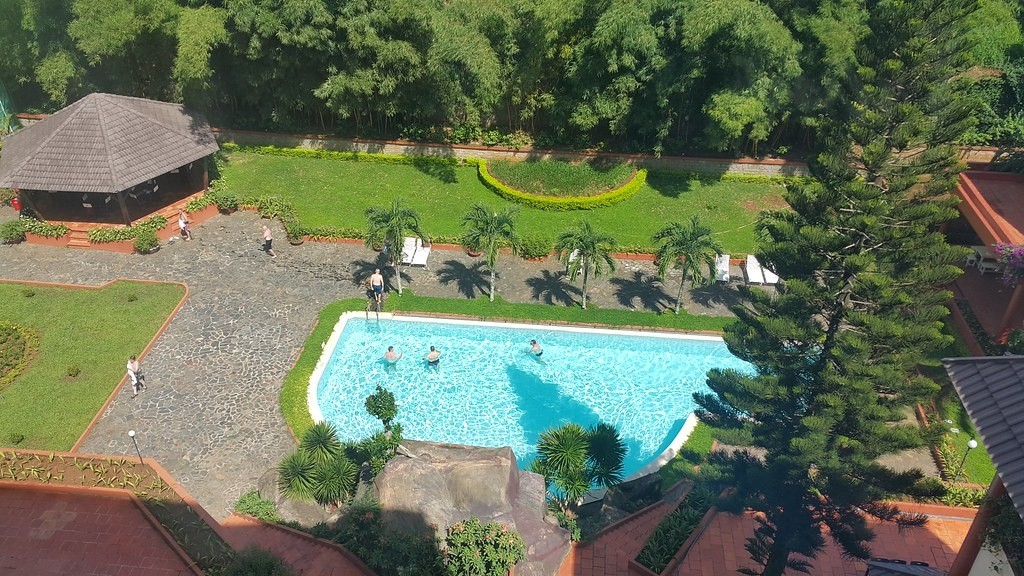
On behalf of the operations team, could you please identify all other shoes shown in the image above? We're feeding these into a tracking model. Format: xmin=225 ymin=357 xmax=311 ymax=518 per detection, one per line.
xmin=143 ymin=386 xmax=146 ymax=392
xmin=272 ymin=256 xmax=277 ymax=258
xmin=131 ymin=394 xmax=138 ymax=398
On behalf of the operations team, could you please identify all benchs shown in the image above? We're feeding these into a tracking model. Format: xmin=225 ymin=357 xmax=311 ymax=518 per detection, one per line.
xmin=970 ymin=246 xmax=985 ymax=253
xmin=979 ymin=263 xmax=996 ymax=275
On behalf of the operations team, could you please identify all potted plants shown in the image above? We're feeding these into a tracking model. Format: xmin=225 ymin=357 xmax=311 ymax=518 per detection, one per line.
xmin=467 ymin=237 xmax=481 ymax=257
xmin=217 ymin=193 xmax=236 ymax=214
xmin=290 ymin=227 xmax=303 ymax=245
xmin=134 ymin=232 xmax=160 ymax=253
xmin=372 ymin=228 xmax=385 ymax=251
xmin=257 ymin=197 xmax=275 ymax=218
xmin=0 ymin=220 xmax=26 ymax=244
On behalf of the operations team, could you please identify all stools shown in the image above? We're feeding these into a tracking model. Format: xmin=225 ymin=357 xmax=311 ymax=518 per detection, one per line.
xmin=963 ymin=255 xmax=976 ymax=266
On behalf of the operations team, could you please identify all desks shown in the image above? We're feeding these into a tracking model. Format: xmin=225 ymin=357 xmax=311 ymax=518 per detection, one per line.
xmin=974 ymin=248 xmax=993 ymax=268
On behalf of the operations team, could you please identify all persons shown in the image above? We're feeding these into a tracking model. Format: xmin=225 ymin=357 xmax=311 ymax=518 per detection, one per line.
xmin=427 ymin=346 xmax=440 ymax=360
xmin=127 ymin=356 xmax=146 ymax=398
xmin=528 ymin=340 xmax=542 ymax=355
xmin=179 ymin=209 xmax=191 ymax=241
xmin=384 ymin=346 xmax=402 ymax=359
xmin=371 ymin=268 xmax=385 ymax=302
xmin=262 ymin=226 xmax=277 ymax=259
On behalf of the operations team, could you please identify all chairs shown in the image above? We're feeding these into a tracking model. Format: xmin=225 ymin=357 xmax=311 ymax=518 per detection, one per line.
xmin=397 ymin=237 xmax=416 ymax=265
xmin=568 ymin=249 xmax=583 ymax=275
xmin=412 ymin=238 xmax=431 ymax=268
xmin=762 ymin=263 xmax=779 ymax=285
xmin=714 ymin=254 xmax=730 ymax=283
xmin=746 ymin=254 xmax=764 ymax=285
